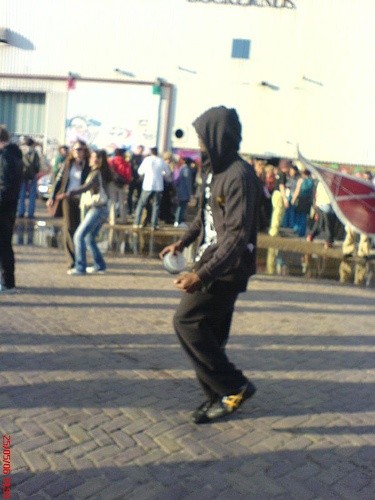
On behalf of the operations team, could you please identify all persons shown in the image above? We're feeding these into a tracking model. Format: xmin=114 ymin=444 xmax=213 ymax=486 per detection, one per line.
xmin=49 ymin=145 xmax=375 ymax=248
xmin=46 ymin=139 xmax=91 ymax=269
xmin=0 ymin=125 xmax=23 ymax=292
xmin=57 ymin=149 xmax=111 ymax=275
xmin=159 ymin=105 xmax=260 ymax=423
xmin=17 ymin=136 xmax=40 ymax=217
xmin=342 ymin=173 xmax=375 ymax=257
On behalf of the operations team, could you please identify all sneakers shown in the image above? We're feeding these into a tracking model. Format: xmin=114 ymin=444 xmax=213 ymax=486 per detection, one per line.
xmin=192 ymin=395 xmax=222 ymax=423
xmin=206 ymin=381 xmax=256 ymax=419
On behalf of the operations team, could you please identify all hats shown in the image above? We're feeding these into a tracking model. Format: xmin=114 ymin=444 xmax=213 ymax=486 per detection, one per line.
xmin=162 ymin=152 xmax=171 ymax=159
xmin=183 ymin=157 xmax=195 ymax=165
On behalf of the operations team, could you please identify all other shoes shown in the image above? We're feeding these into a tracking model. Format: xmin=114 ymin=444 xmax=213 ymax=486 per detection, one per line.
xmin=67 ymin=268 xmax=86 ymax=275
xmin=18 ymin=215 xmax=25 ymax=219
xmin=180 ymin=222 xmax=188 ymax=228
xmin=306 ymin=233 xmax=312 ymax=242
xmin=140 ymin=224 xmax=146 ymax=228
xmin=86 ymin=266 xmax=106 ymax=274
xmin=324 ymin=240 xmax=333 ymax=249
xmin=109 ymin=222 xmax=115 ymax=225
xmin=28 ymin=215 xmax=35 ymax=219
xmin=122 ymin=221 xmax=128 ymax=224
xmin=151 ymin=226 xmax=159 ymax=231
xmin=173 ymin=222 xmax=178 ymax=228
xmin=133 ymin=224 xmax=139 ymax=228
xmin=0 ymin=284 xmax=18 ymax=294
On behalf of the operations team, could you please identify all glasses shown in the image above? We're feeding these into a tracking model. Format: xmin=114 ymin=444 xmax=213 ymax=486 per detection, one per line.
xmin=73 ymin=147 xmax=84 ymax=151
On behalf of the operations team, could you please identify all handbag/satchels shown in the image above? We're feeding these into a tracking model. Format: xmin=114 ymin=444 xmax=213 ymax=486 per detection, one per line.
xmin=48 ymin=199 xmax=63 ymax=217
xmin=84 ymin=170 xmax=108 ymax=207
